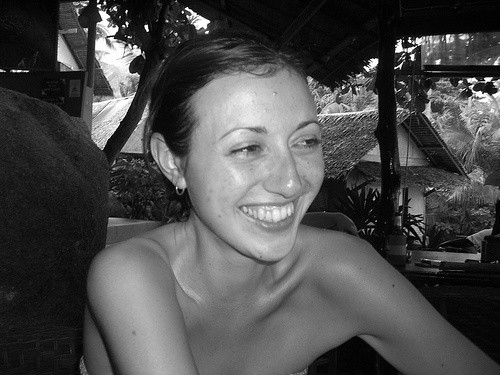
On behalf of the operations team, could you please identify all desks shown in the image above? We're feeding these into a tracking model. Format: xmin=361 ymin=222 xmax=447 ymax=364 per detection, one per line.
xmin=405 ymin=250 xmax=500 ymax=321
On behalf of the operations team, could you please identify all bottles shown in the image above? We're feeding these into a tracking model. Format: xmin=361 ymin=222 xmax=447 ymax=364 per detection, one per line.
xmin=386 ymin=212 xmax=407 ymax=267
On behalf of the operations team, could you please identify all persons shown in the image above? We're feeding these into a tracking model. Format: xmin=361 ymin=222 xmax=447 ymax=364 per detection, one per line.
xmin=79 ymin=32 xmax=500 ymax=375
